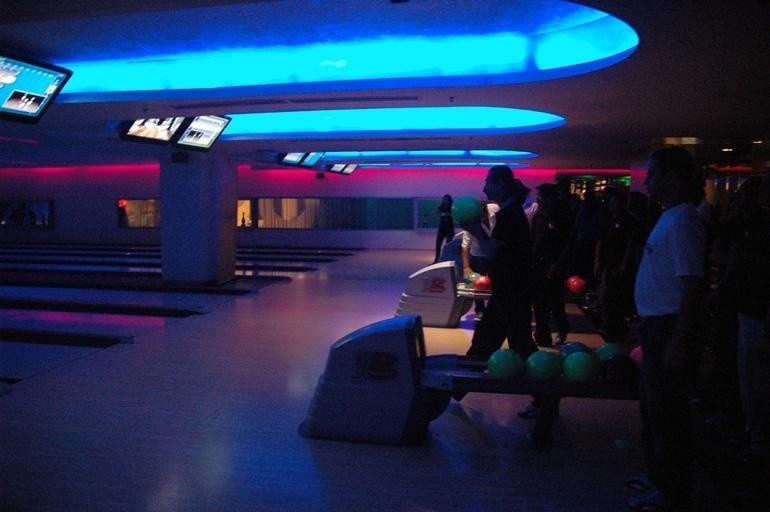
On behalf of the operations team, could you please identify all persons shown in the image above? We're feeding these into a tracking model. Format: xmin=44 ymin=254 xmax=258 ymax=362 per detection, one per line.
xmin=624 ymin=145 xmax=770 ymax=511
xmin=431 ymin=194 xmax=455 ymax=263
xmin=450 ymin=165 xmax=560 ymax=419
xmin=530 ymin=182 xmax=663 ymax=347
xmin=422 ymin=214 xmax=429 ymax=229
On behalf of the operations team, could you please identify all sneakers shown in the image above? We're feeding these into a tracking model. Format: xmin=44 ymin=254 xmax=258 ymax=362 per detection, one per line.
xmin=519 ymin=403 xmax=558 ymax=417
xmin=627 ymin=494 xmax=667 ymax=511
xmin=624 ymin=473 xmax=659 ymax=492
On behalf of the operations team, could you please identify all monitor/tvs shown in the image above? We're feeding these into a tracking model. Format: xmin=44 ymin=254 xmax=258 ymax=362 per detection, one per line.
xmin=0 ymin=44 xmax=73 ymax=125
xmin=119 ymin=116 xmax=186 ymax=147
xmin=278 ymin=151 xmax=359 ymax=175
xmin=173 ymin=111 xmax=232 ymax=153
